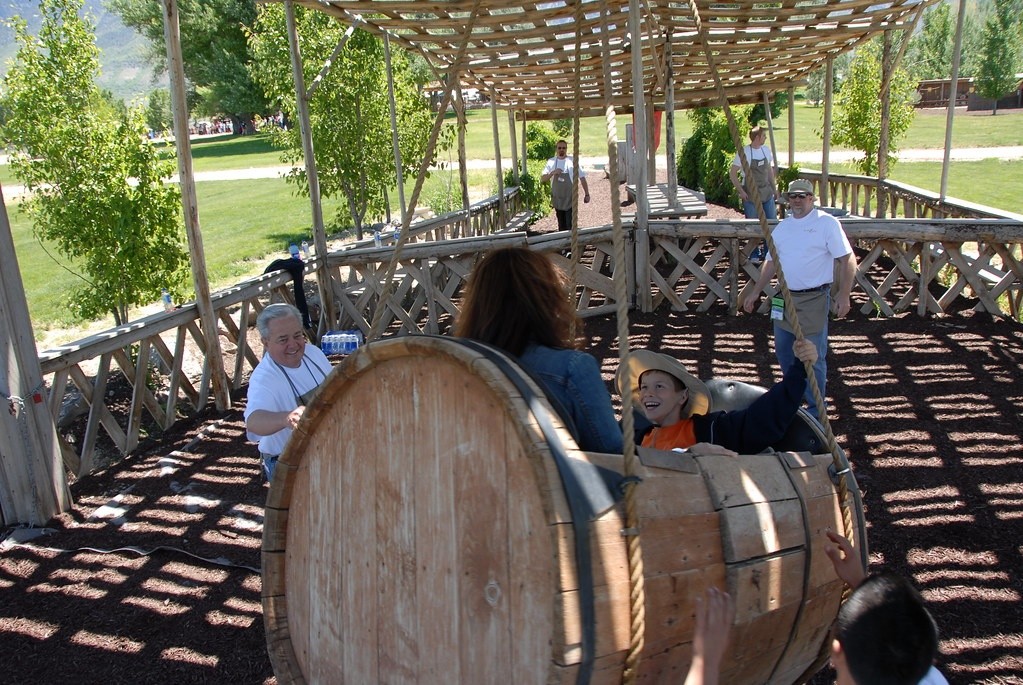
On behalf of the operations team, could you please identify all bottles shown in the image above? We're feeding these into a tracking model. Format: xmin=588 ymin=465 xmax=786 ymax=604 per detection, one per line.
xmin=301 ymin=239 xmax=311 ymax=258
xmin=321 ymin=329 xmax=363 ymax=354
xmin=289 ymin=242 xmax=300 ymax=261
xmin=161 ymin=288 xmax=176 ymax=312
xmin=374 ymin=229 xmax=382 ymax=248
xmin=394 ymin=228 xmax=400 ymax=246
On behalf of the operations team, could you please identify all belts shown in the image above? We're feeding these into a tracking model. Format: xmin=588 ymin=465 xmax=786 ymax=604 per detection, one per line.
xmin=790 ymin=284 xmax=829 ymax=293
xmin=271 ymin=455 xmax=279 ymax=461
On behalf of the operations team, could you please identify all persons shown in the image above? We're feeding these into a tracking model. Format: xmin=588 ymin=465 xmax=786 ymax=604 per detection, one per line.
xmin=614 ymin=340 xmax=818 ymax=455
xmin=731 ymin=126 xmax=778 ymax=219
xmin=149 ymin=109 xmax=289 ymax=137
xmin=449 ymin=247 xmax=738 ymax=457
xmin=683 ymin=528 xmax=948 ymax=685
xmin=541 ymin=140 xmax=590 ymax=231
xmin=743 ymin=179 xmax=857 ymax=418
xmin=243 ymin=303 xmax=333 ymax=483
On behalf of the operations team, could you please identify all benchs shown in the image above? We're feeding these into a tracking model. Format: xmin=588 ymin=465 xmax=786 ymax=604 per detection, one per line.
xmin=490 ymin=210 xmax=534 ymax=235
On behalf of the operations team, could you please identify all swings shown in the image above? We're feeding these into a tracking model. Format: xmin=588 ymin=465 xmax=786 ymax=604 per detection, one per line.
xmin=260 ymin=3 xmax=868 ymax=685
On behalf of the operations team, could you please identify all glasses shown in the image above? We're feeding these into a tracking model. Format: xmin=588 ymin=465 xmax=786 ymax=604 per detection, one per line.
xmin=558 ymin=147 xmax=567 ymax=150
xmin=788 ymin=194 xmax=810 ymax=199
xmin=265 ymin=332 xmax=304 ymax=345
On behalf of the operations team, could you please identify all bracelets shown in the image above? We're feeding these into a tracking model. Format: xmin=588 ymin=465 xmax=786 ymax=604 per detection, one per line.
xmin=585 ymin=194 xmax=588 ymax=196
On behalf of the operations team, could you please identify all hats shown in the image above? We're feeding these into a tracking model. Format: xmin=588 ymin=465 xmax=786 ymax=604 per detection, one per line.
xmin=614 ymin=350 xmax=712 ymax=420
xmin=783 ymin=179 xmax=813 ymax=195
